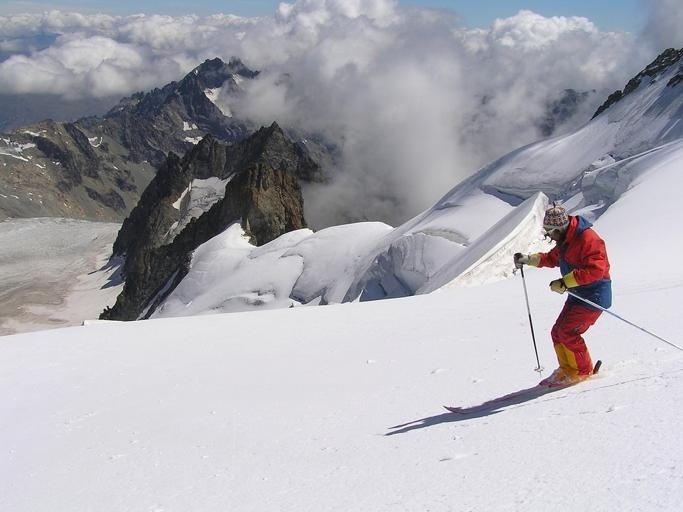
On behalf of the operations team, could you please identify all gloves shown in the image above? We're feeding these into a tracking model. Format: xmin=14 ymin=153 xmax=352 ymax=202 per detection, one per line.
xmin=550 ymin=280 xmax=566 ymax=294
xmin=514 ymin=253 xmax=530 ymax=268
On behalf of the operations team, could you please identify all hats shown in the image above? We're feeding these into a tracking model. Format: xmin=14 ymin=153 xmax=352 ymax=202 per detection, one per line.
xmin=543 ymin=202 xmax=569 ymax=228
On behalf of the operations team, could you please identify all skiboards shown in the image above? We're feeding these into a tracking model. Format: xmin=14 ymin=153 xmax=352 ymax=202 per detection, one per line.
xmin=443 ymin=359 xmax=601 ymax=414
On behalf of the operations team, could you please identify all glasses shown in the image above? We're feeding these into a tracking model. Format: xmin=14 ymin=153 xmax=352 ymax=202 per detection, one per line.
xmin=542 ymin=229 xmax=556 ymax=237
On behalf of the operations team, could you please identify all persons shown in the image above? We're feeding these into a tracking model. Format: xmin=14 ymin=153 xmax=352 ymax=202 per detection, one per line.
xmin=514 ymin=202 xmax=612 ymax=391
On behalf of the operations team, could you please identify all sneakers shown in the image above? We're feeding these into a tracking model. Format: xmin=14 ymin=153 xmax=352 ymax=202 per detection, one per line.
xmin=540 ymin=369 xmax=593 ymax=387
